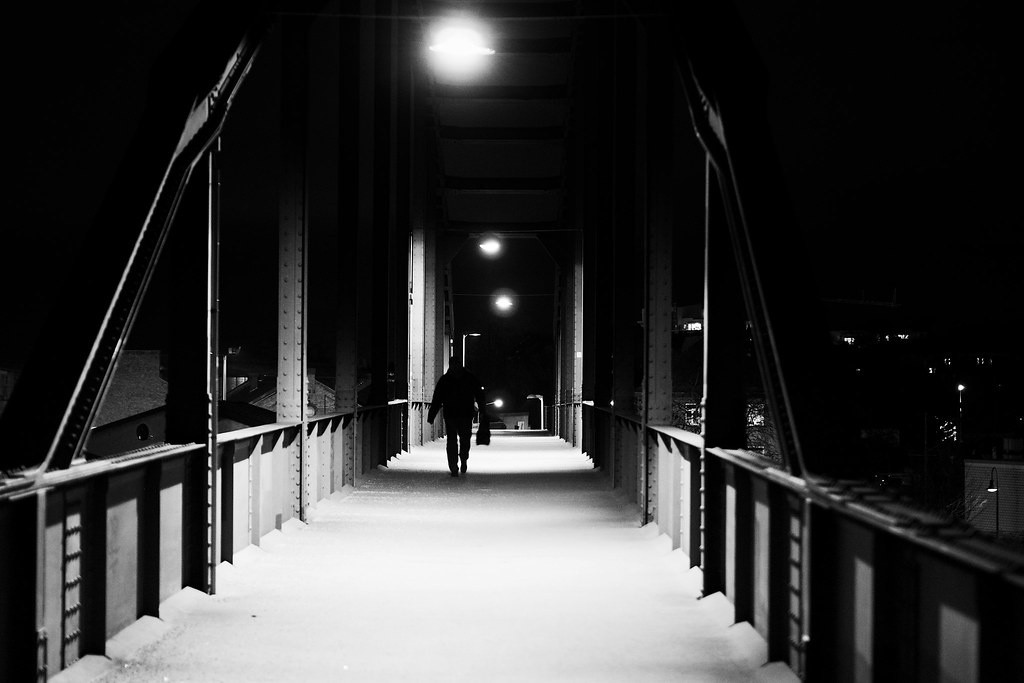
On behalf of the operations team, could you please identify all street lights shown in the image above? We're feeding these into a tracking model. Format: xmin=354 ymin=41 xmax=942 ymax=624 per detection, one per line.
xmin=527 ymin=394 xmax=543 ymax=429
xmin=478 ymin=400 xmax=503 ymax=429
xmin=462 ymin=333 xmax=481 ymax=368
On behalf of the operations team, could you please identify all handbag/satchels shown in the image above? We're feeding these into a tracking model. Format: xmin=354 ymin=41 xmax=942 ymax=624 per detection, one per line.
xmin=474 ymin=413 xmax=492 ymax=445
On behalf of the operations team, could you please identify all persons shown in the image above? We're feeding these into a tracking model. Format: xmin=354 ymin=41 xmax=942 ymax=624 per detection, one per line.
xmin=428 ymin=357 xmax=487 ymax=477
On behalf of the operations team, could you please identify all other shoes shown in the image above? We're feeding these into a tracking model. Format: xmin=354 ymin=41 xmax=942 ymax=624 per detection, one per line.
xmin=460 ymin=465 xmax=467 ymax=473
xmin=450 ymin=469 xmax=458 ymax=477
